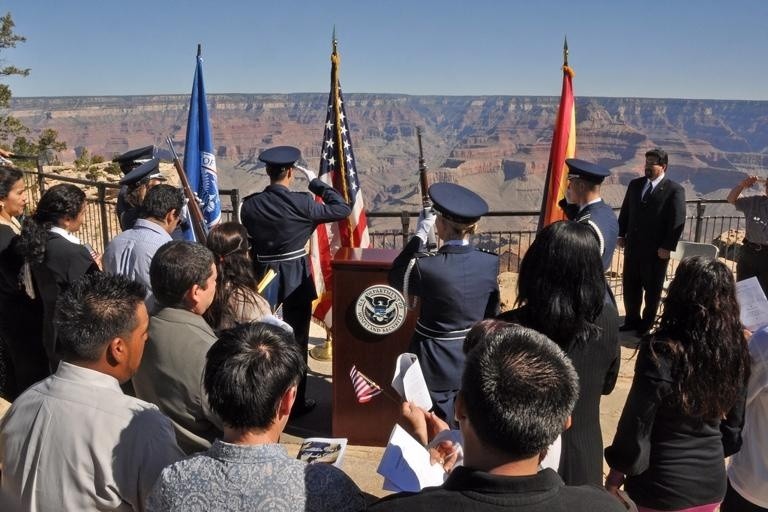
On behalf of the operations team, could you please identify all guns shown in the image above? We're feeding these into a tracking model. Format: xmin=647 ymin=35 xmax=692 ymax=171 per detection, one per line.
xmin=416 ymin=127 xmax=438 ymax=252
xmin=165 ymin=136 xmax=209 ymax=249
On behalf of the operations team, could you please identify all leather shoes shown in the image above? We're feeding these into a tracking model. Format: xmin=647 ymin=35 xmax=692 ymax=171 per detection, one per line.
xmin=636 ymin=325 xmax=648 ymax=336
xmin=619 ymin=324 xmax=636 ymax=330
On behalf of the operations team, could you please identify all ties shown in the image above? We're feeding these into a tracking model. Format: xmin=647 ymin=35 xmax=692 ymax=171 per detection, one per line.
xmin=643 ymin=182 xmax=652 ymax=201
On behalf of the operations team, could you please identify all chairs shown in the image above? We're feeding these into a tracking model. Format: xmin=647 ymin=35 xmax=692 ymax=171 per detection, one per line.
xmin=654 ymin=240 xmax=720 ymax=327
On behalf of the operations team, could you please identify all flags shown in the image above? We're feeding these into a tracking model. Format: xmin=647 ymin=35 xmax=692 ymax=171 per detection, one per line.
xmin=308 ymin=52 xmax=371 ymax=333
xmin=181 ymin=54 xmax=223 ymax=243
xmin=536 ymin=64 xmax=577 ymax=236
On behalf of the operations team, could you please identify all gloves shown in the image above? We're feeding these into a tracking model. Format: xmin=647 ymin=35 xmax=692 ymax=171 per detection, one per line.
xmin=179 ymin=191 xmax=204 ymax=220
xmin=564 ymin=188 xmax=574 ymax=204
xmin=415 ymin=206 xmax=437 ymax=242
xmin=297 ymin=166 xmax=317 ymax=183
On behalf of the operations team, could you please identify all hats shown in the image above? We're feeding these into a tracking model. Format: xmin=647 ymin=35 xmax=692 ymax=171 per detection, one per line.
xmin=564 ymin=158 xmax=612 ymax=183
xmin=428 ymin=183 xmax=488 ymax=224
xmin=119 ymin=157 xmax=167 ymax=184
xmin=112 ymin=145 xmax=157 ymax=164
xmin=258 ymin=146 xmax=300 ymax=167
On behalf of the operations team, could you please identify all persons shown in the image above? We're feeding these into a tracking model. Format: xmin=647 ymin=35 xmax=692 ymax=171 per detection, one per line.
xmin=0 ymin=146 xmax=768 ymax=512
